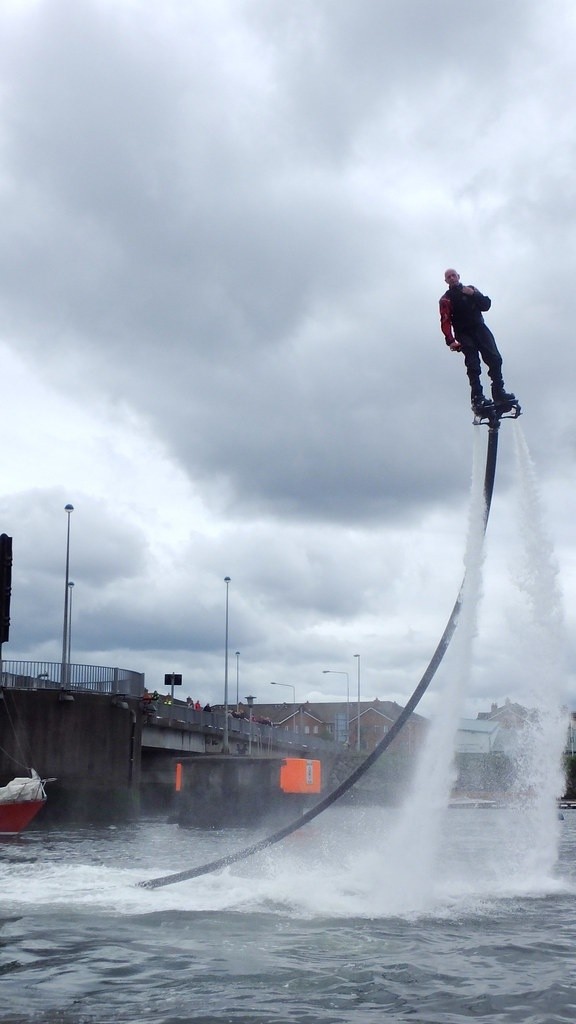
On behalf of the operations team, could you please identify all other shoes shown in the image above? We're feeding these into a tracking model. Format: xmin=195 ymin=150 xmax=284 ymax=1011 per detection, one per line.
xmin=492 ymin=384 xmax=515 ymax=402
xmin=471 ymin=388 xmax=492 ymax=407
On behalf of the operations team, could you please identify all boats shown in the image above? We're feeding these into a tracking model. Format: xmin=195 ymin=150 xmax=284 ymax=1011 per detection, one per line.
xmin=0 ymin=778 xmax=56 ymax=836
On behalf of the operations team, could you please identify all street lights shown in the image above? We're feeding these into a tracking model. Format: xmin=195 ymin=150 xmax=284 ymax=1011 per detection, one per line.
xmin=67 ymin=582 xmax=75 ymax=686
xmin=221 ymin=577 xmax=231 ymax=753
xmin=323 ymin=671 xmax=349 ymax=742
xmin=354 ymin=654 xmax=360 ymax=750
xmin=271 ymin=682 xmax=295 ymax=732
xmin=60 ymin=504 xmax=74 ymax=687
xmin=245 ymin=695 xmax=257 ymax=754
xmin=236 ymin=652 xmax=240 ymax=712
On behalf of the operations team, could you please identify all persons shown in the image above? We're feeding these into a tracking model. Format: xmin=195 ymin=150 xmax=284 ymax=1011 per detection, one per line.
xmin=144 ymin=689 xmax=271 ymax=725
xmin=438 ymin=269 xmax=515 ymax=407
